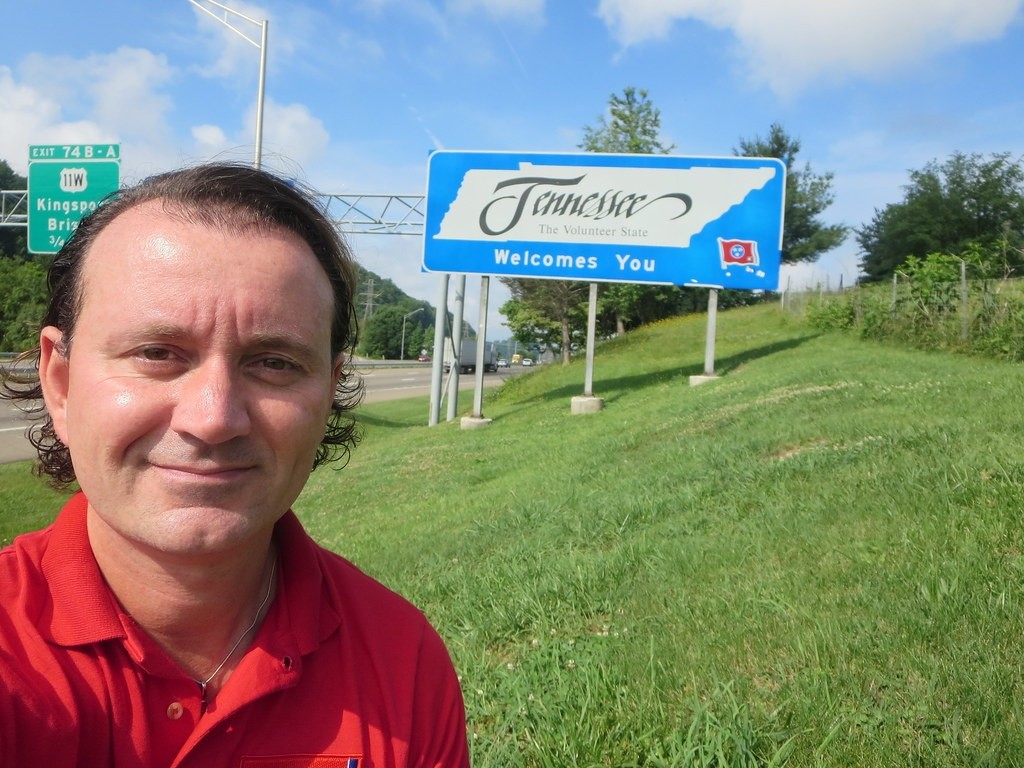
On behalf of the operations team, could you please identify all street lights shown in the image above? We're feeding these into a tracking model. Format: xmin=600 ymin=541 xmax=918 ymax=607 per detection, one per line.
xmin=401 ymin=308 xmax=425 ymax=360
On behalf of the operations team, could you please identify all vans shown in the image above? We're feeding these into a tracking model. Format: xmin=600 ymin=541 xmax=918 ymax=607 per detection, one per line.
xmin=511 ymin=354 xmax=523 ymax=366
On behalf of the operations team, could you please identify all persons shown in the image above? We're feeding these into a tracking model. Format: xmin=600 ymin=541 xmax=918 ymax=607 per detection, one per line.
xmin=0 ymin=161 xmax=469 ymax=768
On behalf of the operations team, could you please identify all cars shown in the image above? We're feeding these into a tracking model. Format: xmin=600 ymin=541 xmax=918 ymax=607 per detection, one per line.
xmin=417 ymin=355 xmax=431 ymax=363
xmin=523 ymin=358 xmax=534 ymax=366
xmin=498 ymin=358 xmax=511 ymax=368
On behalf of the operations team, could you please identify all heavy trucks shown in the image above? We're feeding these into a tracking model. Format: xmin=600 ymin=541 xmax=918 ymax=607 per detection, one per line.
xmin=443 ymin=336 xmax=502 ymax=374
